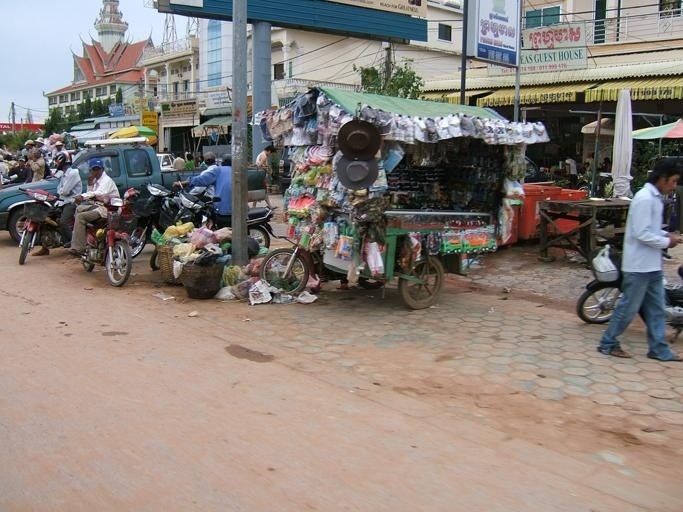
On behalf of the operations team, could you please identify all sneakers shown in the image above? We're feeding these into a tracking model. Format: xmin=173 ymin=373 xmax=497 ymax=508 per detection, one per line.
xmin=32 ymin=248 xmax=50 ymax=256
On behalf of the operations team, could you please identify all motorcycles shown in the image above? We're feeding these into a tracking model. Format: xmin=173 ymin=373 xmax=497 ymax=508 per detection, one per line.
xmin=14 ymin=176 xmax=278 ymax=291
xmin=571 ymin=229 xmax=682 ymax=345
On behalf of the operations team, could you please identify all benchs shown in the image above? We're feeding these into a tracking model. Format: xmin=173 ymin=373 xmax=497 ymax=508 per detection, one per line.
xmin=590 ymin=224 xmax=669 ymax=258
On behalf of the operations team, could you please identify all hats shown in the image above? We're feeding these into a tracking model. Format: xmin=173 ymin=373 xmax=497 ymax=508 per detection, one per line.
xmin=336 ymin=119 xmax=380 ymax=190
xmin=24 ymin=136 xmax=63 ymax=146
xmin=88 ymin=158 xmax=103 ymax=170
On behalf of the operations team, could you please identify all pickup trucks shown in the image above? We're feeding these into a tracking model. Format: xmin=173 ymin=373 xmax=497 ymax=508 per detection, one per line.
xmin=0 ymin=134 xmax=270 ymax=250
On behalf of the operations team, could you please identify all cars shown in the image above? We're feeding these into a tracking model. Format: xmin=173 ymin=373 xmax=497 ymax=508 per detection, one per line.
xmin=153 ymin=150 xmax=177 ymax=171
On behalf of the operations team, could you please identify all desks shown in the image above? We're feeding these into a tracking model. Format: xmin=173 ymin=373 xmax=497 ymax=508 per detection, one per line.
xmin=538 ymin=198 xmax=632 ymax=265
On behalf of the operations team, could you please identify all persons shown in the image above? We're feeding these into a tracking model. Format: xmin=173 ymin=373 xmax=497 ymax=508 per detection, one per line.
xmin=597 ymin=157 xmax=682 ymax=361
xmin=0 ymin=137 xmax=232 ymax=256
xmin=253 ymin=145 xmax=280 ymax=210
xmin=562 ymin=152 xmax=613 ymax=187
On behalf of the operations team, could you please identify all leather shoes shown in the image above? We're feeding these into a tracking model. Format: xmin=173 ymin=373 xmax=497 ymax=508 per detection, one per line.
xmin=598 ymin=345 xmax=632 ymax=358
xmin=647 ymin=351 xmax=683 ymax=361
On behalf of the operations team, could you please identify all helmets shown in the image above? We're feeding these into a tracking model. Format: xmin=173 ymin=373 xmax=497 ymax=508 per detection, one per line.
xmin=54 ymin=151 xmax=72 ymax=168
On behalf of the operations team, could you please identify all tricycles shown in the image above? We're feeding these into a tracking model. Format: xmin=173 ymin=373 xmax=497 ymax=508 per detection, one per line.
xmin=255 ymin=204 xmax=446 ymax=309
